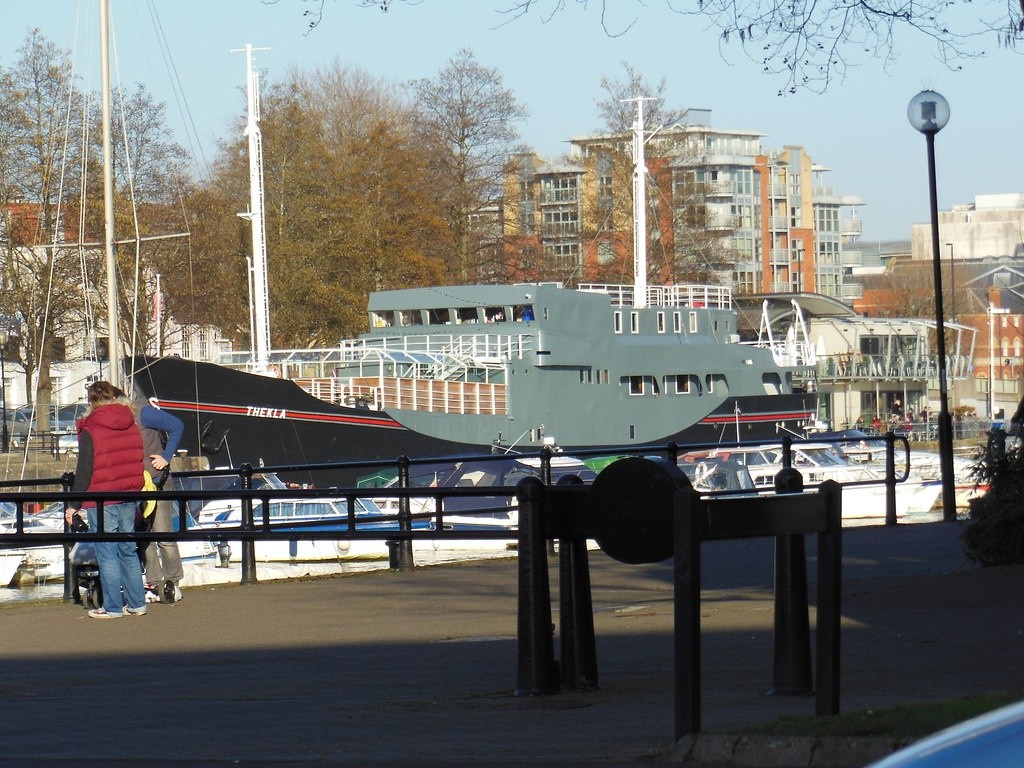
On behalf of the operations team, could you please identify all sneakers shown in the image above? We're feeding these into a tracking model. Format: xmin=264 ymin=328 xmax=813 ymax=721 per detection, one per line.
xmin=88 ymin=608 xmax=115 ymax=619
xmin=145 ymin=591 xmax=160 ymax=603
xmin=122 ymin=604 xmax=147 ymax=615
xmin=174 ymin=588 xmax=182 ymax=602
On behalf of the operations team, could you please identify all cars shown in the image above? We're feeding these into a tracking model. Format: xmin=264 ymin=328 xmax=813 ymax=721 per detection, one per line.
xmin=21 ymin=404 xmax=89 ymax=432
xmin=0 ymin=409 xmax=57 ymax=437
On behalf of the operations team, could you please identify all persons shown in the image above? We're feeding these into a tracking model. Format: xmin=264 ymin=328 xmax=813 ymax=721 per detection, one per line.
xmin=920 ymin=407 xmax=934 ymax=433
xmin=889 ymin=405 xmax=915 ymax=431
xmin=852 ymin=417 xmax=864 ymax=432
xmin=65 ymin=381 xmax=184 ymax=618
xmin=872 ymin=416 xmax=880 ymax=434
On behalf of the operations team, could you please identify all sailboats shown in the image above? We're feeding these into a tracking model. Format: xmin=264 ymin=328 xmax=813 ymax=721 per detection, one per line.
xmin=0 ymin=0 xmax=225 ymax=586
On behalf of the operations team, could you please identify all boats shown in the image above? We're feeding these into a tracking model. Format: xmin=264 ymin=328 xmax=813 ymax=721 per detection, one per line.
xmin=362 ymin=428 xmax=599 ymax=532
xmin=118 ymin=42 xmax=822 ymax=489
xmin=675 ymin=401 xmax=992 ymax=518
xmin=197 ymin=458 xmax=437 ymax=559
xmin=34 ymin=503 xmax=68 ymax=529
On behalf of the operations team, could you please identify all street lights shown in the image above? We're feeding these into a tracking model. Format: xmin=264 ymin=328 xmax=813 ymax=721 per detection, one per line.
xmin=945 ymin=243 xmax=956 ymax=352
xmin=908 ymin=92 xmax=959 ymax=519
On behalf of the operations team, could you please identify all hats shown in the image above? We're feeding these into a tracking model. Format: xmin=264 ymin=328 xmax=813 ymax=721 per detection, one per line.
xmin=136 ymin=470 xmax=157 ymax=519
